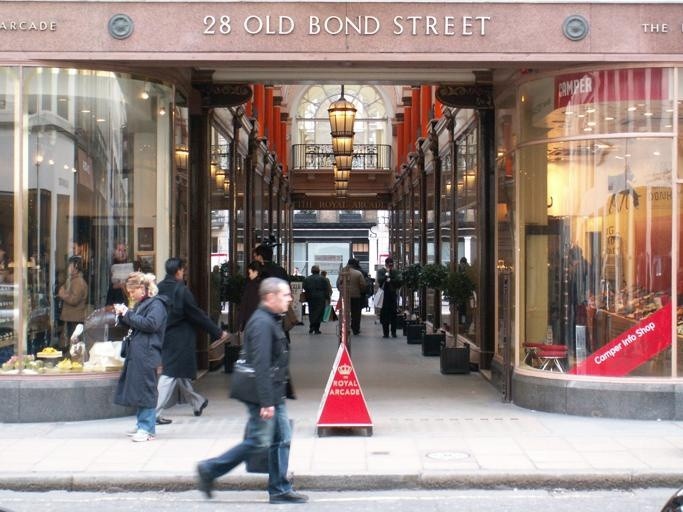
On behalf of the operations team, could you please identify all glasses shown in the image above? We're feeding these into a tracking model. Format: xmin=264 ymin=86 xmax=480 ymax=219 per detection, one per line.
xmin=387 ymin=264 xmax=393 ymax=267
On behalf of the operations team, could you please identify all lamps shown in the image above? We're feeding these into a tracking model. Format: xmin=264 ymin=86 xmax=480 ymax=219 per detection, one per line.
xmin=462 ymin=170 xmax=475 ymax=190
xmin=456 ymin=179 xmax=463 ymax=193
xmin=175 ymin=144 xmax=189 ymax=171
xmin=223 ymin=176 xmax=230 ymax=194
xmin=327 ymin=84 xmax=356 ymax=138
xmin=332 ymin=162 xmax=350 ymax=181
xmin=496 ymin=146 xmax=505 ymax=157
xmin=215 ymin=167 xmax=225 ymax=189
xmin=445 ymin=181 xmax=452 ymax=192
xmin=332 ymin=137 xmax=352 ymax=156
xmin=334 ymin=156 xmax=352 ymax=170
xmin=336 ymin=190 xmax=347 ymax=199
xmin=209 ymin=159 xmax=216 ymax=177
xmin=334 ymin=181 xmax=348 ymax=191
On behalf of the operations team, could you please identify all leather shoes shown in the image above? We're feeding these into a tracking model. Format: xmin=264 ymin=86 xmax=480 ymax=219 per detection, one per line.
xmin=156 ymin=417 xmax=172 ymax=424
xmin=309 ymin=329 xmax=313 ymax=333
xmin=315 ymin=331 xmax=322 ymax=334
xmin=269 ymin=490 xmax=309 ymax=504
xmin=287 ymin=394 xmax=296 ymax=399
xmin=194 ymin=398 xmax=208 ymax=416
xmin=197 ymin=464 xmax=213 ymax=499
xmin=353 ymin=331 xmax=360 ymax=335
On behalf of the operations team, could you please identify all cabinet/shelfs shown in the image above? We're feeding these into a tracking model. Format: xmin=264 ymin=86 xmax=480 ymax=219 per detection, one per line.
xmin=584 ymin=294 xmax=682 ymax=376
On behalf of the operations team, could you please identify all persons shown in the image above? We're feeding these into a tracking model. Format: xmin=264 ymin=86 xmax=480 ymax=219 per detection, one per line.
xmin=252 ymin=244 xmax=292 ymax=288
xmin=198 ymin=278 xmax=308 ymax=505
xmin=570 ymin=247 xmax=595 ymax=356
xmin=105 ymin=242 xmax=142 ymax=340
xmin=337 ymin=259 xmax=367 ymax=335
xmin=114 ymin=273 xmax=171 ymax=442
xmin=351 ymin=259 xmax=375 ymax=329
xmin=239 ymin=261 xmax=296 ymax=399
xmin=58 ymin=256 xmax=89 ymax=351
xmin=153 ymin=257 xmax=227 ymax=423
xmin=378 ymin=258 xmax=401 ymax=337
xmin=302 ymin=265 xmax=330 ymax=334
xmin=321 ymin=271 xmax=332 ymax=322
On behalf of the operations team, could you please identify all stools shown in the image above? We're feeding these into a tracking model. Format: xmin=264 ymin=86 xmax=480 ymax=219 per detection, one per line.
xmin=522 ymin=342 xmax=543 ymax=367
xmin=541 ymin=345 xmax=567 ymax=371
xmin=536 ymin=351 xmax=566 ymax=373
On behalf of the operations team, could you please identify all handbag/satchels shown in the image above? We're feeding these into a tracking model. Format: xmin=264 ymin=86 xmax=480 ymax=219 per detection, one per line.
xmin=329 ymin=306 xmax=339 ymax=322
xmin=121 ymin=327 xmax=134 ymax=358
xmin=322 ymin=307 xmax=331 ymax=322
xmin=243 ymin=417 xmax=293 ymax=473
xmin=229 ymin=332 xmax=284 ymax=406
xmin=299 ymin=288 xmax=308 ymax=302
xmin=373 ymin=282 xmax=386 ymax=308
xmin=284 ymin=305 xmax=297 ymax=331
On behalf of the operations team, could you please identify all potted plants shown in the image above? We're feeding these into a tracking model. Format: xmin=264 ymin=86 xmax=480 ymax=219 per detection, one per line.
xmin=391 ymin=267 xmax=408 ymax=329
xmin=401 ymin=266 xmax=422 ymax=336
xmin=217 ymin=261 xmax=245 ymax=373
xmin=406 ymin=264 xmax=428 ymax=344
xmin=440 ymin=266 xmax=476 ymax=375
xmin=416 ymin=263 xmax=447 ymax=357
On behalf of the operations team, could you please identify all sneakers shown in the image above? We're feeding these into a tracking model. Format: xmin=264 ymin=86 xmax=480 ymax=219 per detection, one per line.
xmin=124 ymin=426 xmax=138 ymax=436
xmin=132 ymin=430 xmax=154 ymax=442
